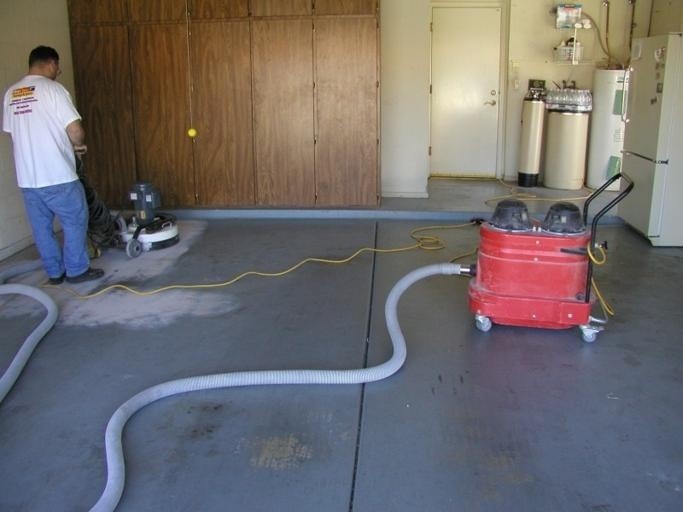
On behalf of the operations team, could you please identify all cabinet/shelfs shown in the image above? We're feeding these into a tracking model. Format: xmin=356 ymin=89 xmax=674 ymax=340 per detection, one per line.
xmin=66 ymin=0 xmax=383 ymax=209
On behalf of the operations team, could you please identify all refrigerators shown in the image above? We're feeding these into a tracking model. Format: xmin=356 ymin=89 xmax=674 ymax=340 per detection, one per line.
xmin=614 ymin=32 xmax=683 ymax=247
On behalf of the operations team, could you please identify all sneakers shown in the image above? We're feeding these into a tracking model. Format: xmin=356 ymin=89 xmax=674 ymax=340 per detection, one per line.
xmin=66 ymin=268 xmax=104 ymax=283
xmin=48 ymin=271 xmax=66 ymax=286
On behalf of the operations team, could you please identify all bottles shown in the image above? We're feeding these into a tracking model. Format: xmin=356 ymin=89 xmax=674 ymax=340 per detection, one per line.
xmin=546 ymin=87 xmax=592 ymax=113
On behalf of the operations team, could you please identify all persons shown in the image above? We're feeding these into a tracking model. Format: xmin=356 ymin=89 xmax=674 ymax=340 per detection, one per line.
xmin=1 ymin=44 xmax=105 ymax=287
